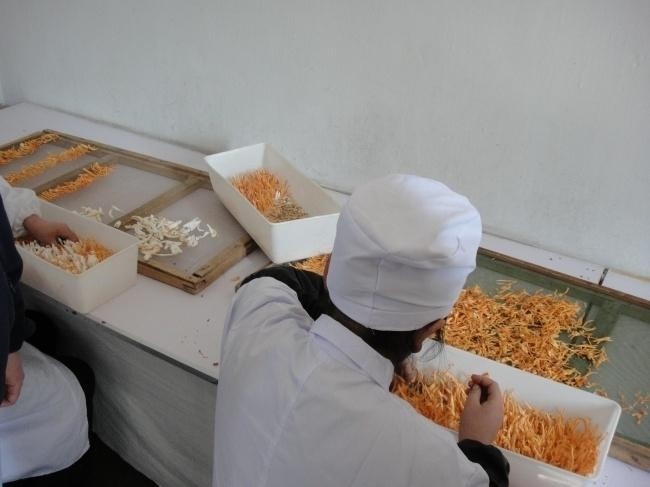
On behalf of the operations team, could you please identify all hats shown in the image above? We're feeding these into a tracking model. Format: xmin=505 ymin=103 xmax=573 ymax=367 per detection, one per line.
xmin=326 ymin=175 xmax=482 ymax=332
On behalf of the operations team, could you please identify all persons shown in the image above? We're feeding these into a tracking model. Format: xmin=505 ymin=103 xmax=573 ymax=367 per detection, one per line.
xmin=1 ymin=172 xmax=95 ymax=485
xmin=216 ymin=175 xmax=511 ymax=486
xmin=2 ymin=198 xmax=24 ymax=486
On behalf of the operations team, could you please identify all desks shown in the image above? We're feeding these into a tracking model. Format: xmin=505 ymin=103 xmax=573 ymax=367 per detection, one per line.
xmin=1 ymin=100 xmax=650 ymax=487
